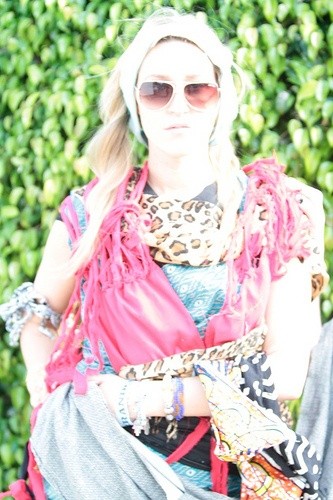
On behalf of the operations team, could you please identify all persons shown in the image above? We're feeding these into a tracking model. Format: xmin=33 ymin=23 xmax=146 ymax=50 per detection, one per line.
xmin=14 ymin=6 xmax=327 ymax=500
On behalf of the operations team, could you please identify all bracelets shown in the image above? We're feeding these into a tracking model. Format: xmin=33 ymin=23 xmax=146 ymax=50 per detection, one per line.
xmin=117 ymin=373 xmax=185 ymax=437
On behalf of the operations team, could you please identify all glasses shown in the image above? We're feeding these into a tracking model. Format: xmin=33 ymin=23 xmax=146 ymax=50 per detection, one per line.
xmin=135 ymin=80 xmax=221 ymax=113
